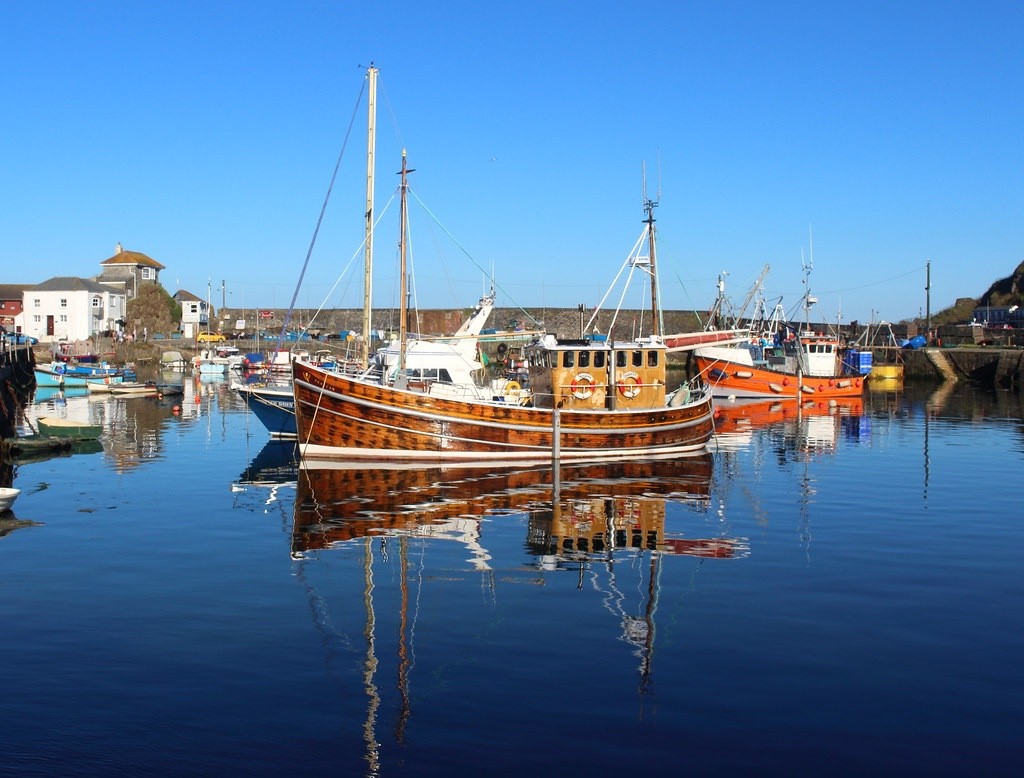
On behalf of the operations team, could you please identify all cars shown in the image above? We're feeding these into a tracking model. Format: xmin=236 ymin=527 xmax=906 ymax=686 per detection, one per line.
xmin=197 ymin=331 xmax=226 ymax=343
xmin=1 ymin=332 xmax=38 ymax=345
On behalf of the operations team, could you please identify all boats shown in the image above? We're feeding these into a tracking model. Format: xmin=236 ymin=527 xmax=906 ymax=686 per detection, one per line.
xmin=35 ymin=386 xmax=91 ymax=407
xmin=36 ymin=360 xmax=137 ymax=382
xmin=37 ymin=416 xmax=104 ymax=440
xmin=712 ymin=395 xmax=863 ymax=455
xmin=49 ymin=339 xmax=101 ymax=363
xmin=159 ymin=352 xmax=186 ymax=367
xmin=86 ymin=382 xmax=184 ymax=398
xmin=34 ymin=362 xmax=122 ymax=387
xmin=693 ymin=261 xmax=863 ymax=398
xmin=0 ymin=487 xmax=21 ymax=512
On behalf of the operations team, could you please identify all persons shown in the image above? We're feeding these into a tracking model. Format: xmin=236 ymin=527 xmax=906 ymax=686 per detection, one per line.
xmin=60 ymin=379 xmax=65 ymax=398
xmin=117 ymin=367 xmax=120 ymax=374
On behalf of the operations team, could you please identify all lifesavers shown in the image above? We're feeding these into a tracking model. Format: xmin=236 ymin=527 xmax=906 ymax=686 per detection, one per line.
xmin=570 ymin=373 xmax=596 ymax=400
xmin=506 ymin=381 xmax=521 ymax=395
xmin=619 ymin=372 xmax=643 ymax=398
xmin=497 ymin=353 xmax=506 ymax=362
xmin=497 ymin=343 xmax=507 ymax=353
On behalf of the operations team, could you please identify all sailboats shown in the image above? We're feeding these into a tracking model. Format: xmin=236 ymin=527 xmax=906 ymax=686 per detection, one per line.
xmin=232 ymin=436 xmax=493 ymax=773
xmin=291 ymin=449 xmax=715 ymax=778
xmin=190 ymin=277 xmax=246 ymax=373
xmin=230 ymin=61 xmax=534 ymax=438
xmin=290 ymin=147 xmax=715 ymax=460
